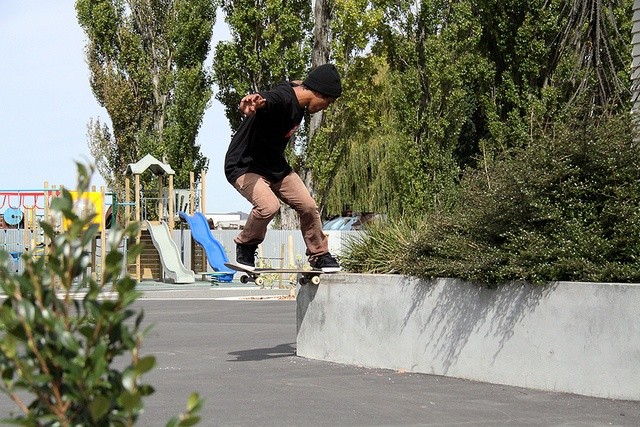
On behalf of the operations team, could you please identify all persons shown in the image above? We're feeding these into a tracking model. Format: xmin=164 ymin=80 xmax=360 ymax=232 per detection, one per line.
xmin=224 ymin=63 xmax=341 ymax=275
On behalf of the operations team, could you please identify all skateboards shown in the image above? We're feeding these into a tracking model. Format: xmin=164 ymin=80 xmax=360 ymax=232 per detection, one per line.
xmin=224 ymin=263 xmax=325 ymax=285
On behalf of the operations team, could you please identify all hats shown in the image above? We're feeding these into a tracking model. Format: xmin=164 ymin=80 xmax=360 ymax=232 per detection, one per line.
xmin=303 ymin=64 xmax=342 ymax=98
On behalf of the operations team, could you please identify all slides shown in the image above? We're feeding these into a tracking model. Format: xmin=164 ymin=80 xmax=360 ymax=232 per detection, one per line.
xmin=177 ymin=211 xmax=236 ymax=282
xmin=145 ymin=220 xmax=196 ymax=285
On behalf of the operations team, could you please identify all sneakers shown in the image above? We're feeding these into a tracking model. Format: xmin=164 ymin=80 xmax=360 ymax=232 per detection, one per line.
xmin=234 ymin=243 xmax=259 ymax=271
xmin=310 ymin=252 xmax=341 ymax=272
